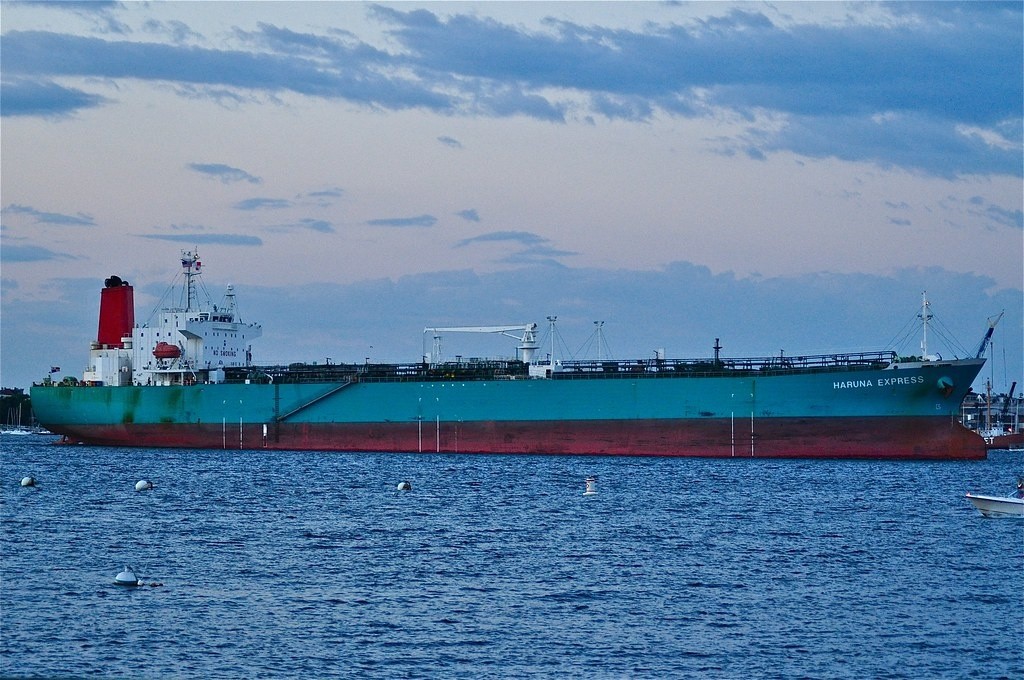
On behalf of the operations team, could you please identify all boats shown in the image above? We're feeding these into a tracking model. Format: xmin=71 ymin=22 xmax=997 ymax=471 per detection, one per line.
xmin=29 ymin=239 xmax=1003 ymax=461
xmin=965 ymin=481 xmax=1024 ymax=518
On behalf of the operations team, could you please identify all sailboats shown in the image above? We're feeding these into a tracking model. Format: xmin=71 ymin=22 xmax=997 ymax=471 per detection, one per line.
xmin=0 ymin=403 xmax=55 ymax=436
xmin=962 ymin=377 xmax=1024 ymax=452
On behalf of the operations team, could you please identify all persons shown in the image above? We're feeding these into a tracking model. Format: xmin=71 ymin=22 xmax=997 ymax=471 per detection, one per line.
xmin=147 ymin=377 xmax=151 ymax=386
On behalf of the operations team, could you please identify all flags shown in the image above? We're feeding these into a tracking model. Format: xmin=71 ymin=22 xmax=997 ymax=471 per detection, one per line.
xmin=183 ymin=261 xmax=192 ymax=267
xmin=51 ymin=366 xmax=61 ymax=374
xmin=196 ymin=262 xmax=201 ymax=270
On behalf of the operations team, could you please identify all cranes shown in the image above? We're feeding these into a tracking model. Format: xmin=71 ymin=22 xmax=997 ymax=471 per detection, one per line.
xmin=422 ymin=322 xmax=542 ymax=364
